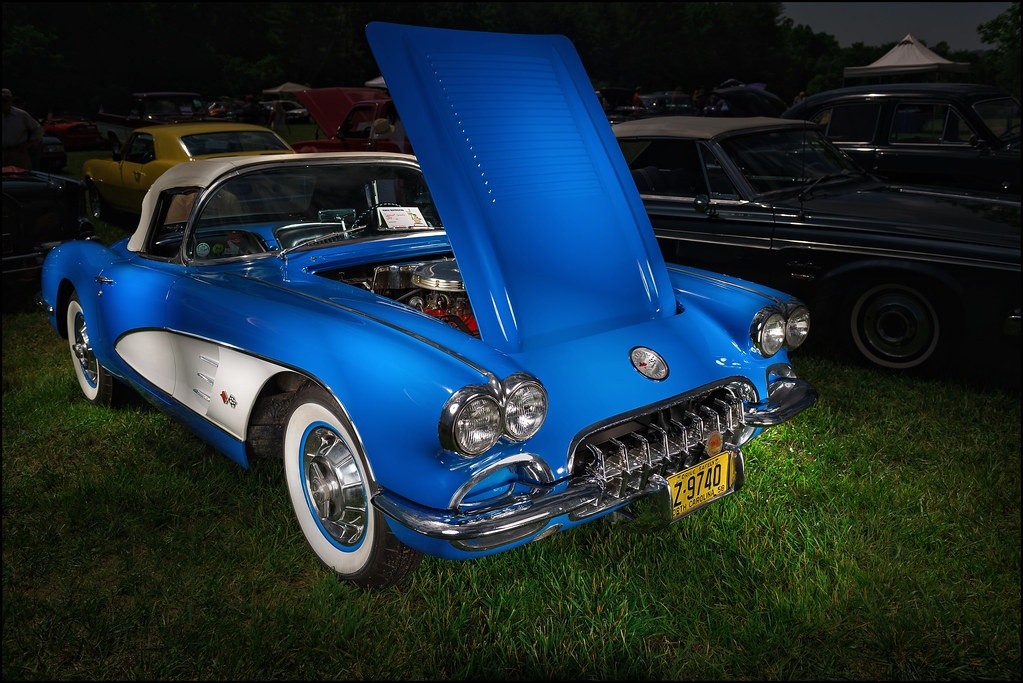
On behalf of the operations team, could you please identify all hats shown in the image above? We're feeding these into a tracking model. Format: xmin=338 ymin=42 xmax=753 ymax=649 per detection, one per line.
xmin=370 ymin=118 xmax=395 ymax=140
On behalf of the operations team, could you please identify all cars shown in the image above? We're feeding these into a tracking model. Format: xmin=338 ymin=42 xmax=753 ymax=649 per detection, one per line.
xmin=593 ymin=81 xmax=787 ymax=124
xmin=39 ymin=90 xmax=310 ymax=151
xmin=31 ymin=22 xmax=822 ymax=589
xmin=82 ymin=121 xmax=316 ymax=223
xmin=0 ymin=164 xmax=98 ymax=289
xmin=778 ymin=83 xmax=1023 ymax=205
xmin=289 ymin=86 xmax=416 ymax=154
xmin=611 ymin=114 xmax=1023 ymax=374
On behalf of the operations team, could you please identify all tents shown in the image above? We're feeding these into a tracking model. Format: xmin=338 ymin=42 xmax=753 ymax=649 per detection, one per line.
xmin=842 ymin=33 xmax=970 ymax=139
xmin=365 ymin=76 xmax=388 ymax=88
xmin=263 ymin=81 xmax=312 ymax=94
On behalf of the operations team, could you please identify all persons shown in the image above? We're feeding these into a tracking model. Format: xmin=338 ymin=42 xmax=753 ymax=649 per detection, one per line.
xmin=1 ymin=89 xmax=43 ymax=169
xmin=267 ymin=101 xmax=291 ymax=139
xmin=793 ymin=92 xmax=806 ymax=105
xmin=633 ymin=87 xmax=642 ymax=105
xmin=692 ymin=87 xmax=719 ymax=108
